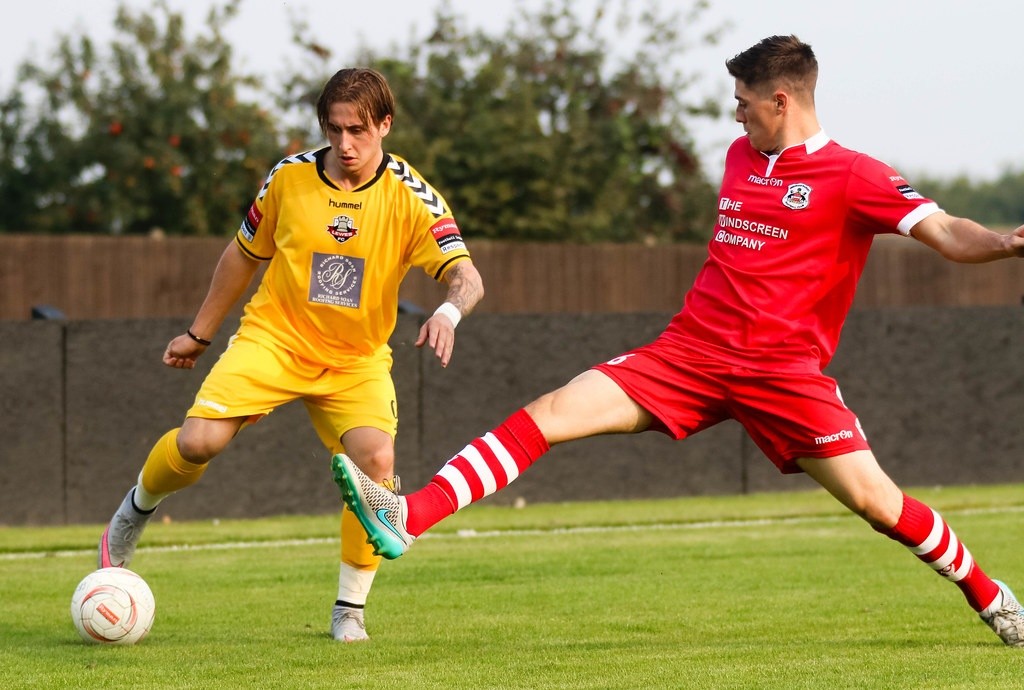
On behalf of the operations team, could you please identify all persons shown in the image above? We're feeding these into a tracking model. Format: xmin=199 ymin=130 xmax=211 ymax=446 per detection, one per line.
xmin=328 ymin=36 xmax=1023 ymax=648
xmin=97 ymin=69 xmax=486 ymax=648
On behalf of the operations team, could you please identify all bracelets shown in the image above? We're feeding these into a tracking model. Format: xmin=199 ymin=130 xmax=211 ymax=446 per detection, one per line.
xmin=431 ymin=302 xmax=461 ymax=329
xmin=187 ymin=330 xmax=212 ymax=347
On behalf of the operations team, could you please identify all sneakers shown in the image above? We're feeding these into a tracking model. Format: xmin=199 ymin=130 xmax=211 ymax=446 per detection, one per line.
xmin=331 ymin=454 xmax=414 ymax=560
xmin=98 ymin=486 xmax=158 ymax=568
xmin=332 ymin=611 xmax=369 ymax=641
xmin=979 ymin=580 xmax=1024 ymax=648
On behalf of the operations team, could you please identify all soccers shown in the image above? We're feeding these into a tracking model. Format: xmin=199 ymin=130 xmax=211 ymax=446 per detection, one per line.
xmin=69 ymin=567 xmax=155 ymax=647
